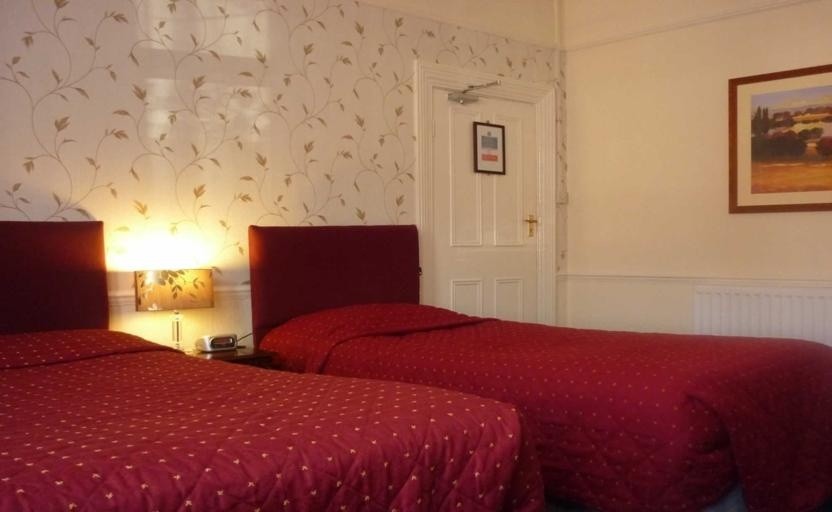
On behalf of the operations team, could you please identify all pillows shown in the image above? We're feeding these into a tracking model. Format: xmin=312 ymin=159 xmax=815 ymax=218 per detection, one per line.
xmin=260 ymin=303 xmax=501 ymax=374
xmin=0 ymin=328 xmax=182 ymax=371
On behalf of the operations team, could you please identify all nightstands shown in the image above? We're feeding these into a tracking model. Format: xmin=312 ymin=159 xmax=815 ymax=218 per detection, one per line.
xmin=165 ymin=337 xmax=279 ymax=370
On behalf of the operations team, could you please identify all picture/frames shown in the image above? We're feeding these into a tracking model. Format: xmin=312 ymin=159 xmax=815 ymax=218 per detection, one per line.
xmin=472 ymin=120 xmax=507 ymax=175
xmin=727 ymin=63 xmax=832 ymax=214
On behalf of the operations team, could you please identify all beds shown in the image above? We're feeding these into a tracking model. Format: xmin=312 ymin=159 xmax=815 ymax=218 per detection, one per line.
xmin=0 ymin=219 xmax=520 ymax=512
xmin=247 ymin=223 xmax=832 ymax=511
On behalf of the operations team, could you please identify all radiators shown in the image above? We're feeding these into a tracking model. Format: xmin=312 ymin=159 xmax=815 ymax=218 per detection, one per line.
xmin=691 ymin=284 xmax=832 ymax=349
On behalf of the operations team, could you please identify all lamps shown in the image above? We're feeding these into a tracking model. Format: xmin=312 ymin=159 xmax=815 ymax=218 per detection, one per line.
xmin=131 ymin=267 xmax=215 ymax=350
